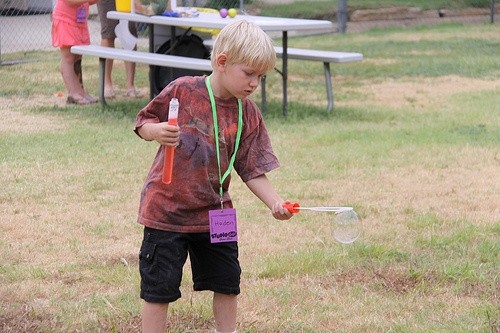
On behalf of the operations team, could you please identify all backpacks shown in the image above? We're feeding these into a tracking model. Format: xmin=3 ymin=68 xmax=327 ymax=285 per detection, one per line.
xmin=149 ymin=27 xmax=212 ymax=101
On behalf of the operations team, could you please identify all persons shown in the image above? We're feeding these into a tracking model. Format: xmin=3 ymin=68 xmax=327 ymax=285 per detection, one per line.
xmin=95 ymin=0 xmax=145 ymax=100
xmin=51 ymin=0 xmax=99 ymax=105
xmin=131 ymin=19 xmax=293 ymax=333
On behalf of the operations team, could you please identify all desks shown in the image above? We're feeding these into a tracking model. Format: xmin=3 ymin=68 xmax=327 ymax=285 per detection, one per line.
xmin=107 ymin=6 xmax=332 ymax=118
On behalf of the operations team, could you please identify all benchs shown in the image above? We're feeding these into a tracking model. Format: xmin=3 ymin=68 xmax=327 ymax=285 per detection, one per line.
xmin=202 ymin=39 xmax=364 ymax=116
xmin=70 ymin=44 xmax=268 ymax=116
